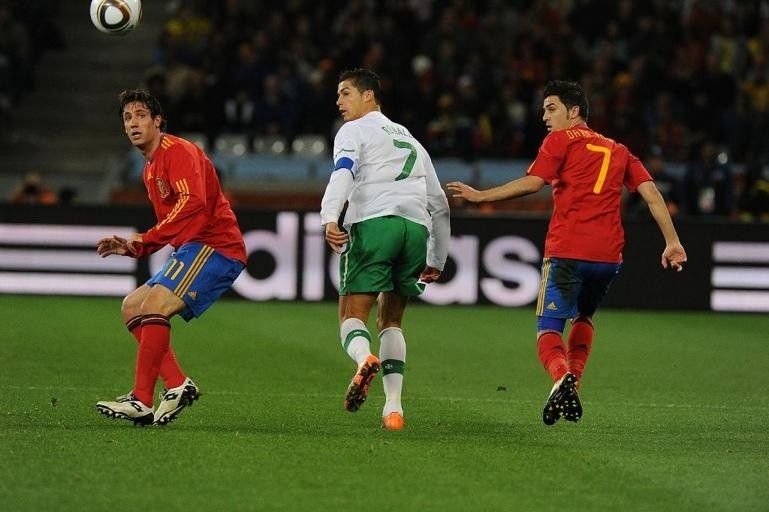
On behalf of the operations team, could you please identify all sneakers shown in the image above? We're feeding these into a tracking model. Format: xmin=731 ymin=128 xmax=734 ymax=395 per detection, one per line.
xmin=382 ymin=412 xmax=404 ymax=430
xmin=96 ymin=376 xmax=199 ymax=425
xmin=543 ymin=371 xmax=583 ymax=426
xmin=343 ymin=355 xmax=381 ymax=412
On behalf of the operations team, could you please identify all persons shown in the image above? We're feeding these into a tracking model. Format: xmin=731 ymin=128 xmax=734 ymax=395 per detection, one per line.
xmin=620 ymin=138 xmax=769 ymax=226
xmin=145 ymin=0 xmax=769 ymax=162
xmin=96 ymin=90 xmax=248 ymax=426
xmin=446 ymin=80 xmax=687 ymax=425
xmin=9 ymin=172 xmax=58 ymax=205
xmin=320 ymin=69 xmax=450 ymax=430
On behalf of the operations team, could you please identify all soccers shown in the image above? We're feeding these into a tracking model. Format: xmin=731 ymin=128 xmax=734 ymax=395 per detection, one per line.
xmin=90 ymin=0 xmax=142 ymax=35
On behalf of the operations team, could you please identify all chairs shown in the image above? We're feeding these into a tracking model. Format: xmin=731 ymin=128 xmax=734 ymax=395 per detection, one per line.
xmin=0 ymin=0 xmax=767 ymax=207
